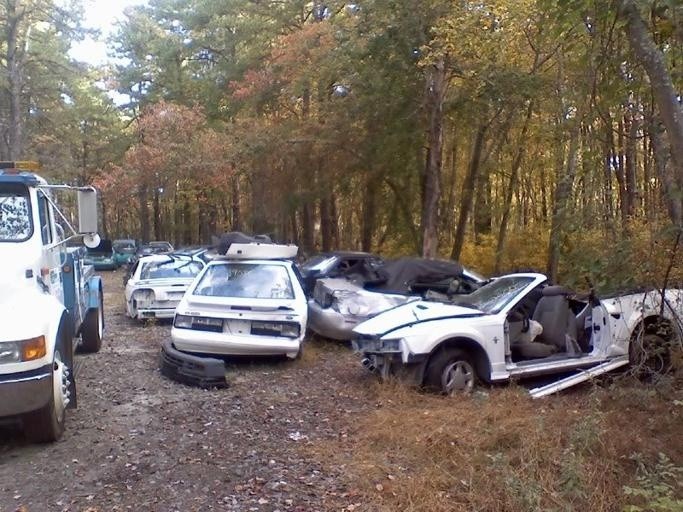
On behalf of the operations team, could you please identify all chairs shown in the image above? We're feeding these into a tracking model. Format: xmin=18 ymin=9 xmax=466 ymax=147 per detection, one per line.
xmin=510 ymin=286 xmax=571 ymax=361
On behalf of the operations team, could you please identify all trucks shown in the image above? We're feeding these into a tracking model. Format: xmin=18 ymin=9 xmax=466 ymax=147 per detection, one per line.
xmin=0 ymin=162 xmax=104 ymax=444
